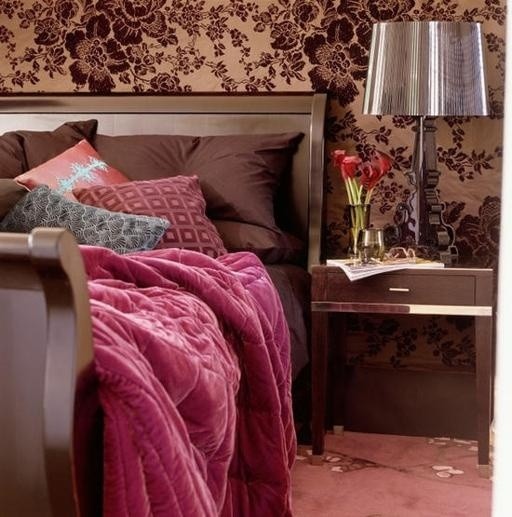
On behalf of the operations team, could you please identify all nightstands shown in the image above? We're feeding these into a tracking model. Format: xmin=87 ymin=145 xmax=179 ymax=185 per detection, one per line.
xmin=309 ymin=263 xmax=495 ymax=463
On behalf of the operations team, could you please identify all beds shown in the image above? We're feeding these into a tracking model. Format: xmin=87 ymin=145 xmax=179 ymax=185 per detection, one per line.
xmin=1 ymin=91 xmax=329 ymax=517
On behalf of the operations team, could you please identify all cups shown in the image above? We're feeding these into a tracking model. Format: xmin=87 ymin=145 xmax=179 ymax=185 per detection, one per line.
xmin=361 ymin=229 xmax=385 ymax=262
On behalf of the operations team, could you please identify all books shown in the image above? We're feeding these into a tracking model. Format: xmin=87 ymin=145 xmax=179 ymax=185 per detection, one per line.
xmin=326 ymin=257 xmax=444 ymax=282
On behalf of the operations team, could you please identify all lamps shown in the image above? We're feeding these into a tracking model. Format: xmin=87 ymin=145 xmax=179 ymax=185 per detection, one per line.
xmin=362 ymin=19 xmax=490 ymax=263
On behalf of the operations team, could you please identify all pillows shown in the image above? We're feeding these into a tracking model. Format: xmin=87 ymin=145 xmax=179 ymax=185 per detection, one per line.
xmin=72 ymin=175 xmax=230 ymax=259
xmin=0 ymin=177 xmax=25 ymax=217
xmin=96 ymin=133 xmax=305 ymax=232
xmin=0 ymin=118 xmax=98 ymax=177
xmin=213 ymin=219 xmax=292 ymax=259
xmin=1 ymin=183 xmax=171 ymax=253
xmin=13 ymin=139 xmax=130 ymax=204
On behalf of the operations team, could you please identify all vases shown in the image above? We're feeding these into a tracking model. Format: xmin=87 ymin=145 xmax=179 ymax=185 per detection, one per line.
xmin=344 ymin=204 xmax=370 ymax=265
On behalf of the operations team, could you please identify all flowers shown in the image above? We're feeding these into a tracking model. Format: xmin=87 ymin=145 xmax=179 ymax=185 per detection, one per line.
xmin=330 ymin=146 xmax=395 ymax=249
